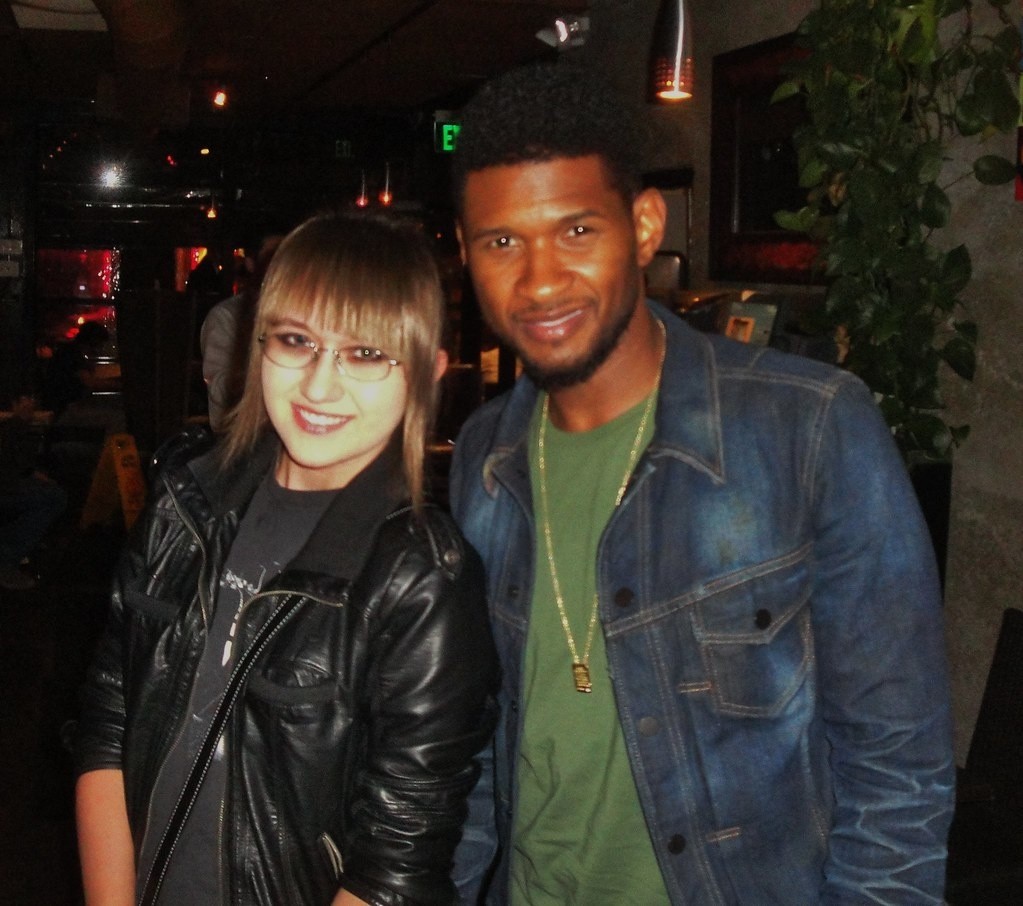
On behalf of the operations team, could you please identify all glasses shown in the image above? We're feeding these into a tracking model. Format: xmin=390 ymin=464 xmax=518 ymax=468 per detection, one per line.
xmin=257 ymin=323 xmax=401 ymax=382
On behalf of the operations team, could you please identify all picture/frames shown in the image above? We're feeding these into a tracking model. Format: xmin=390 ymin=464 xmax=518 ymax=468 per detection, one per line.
xmin=718 ymin=302 xmax=778 ymax=348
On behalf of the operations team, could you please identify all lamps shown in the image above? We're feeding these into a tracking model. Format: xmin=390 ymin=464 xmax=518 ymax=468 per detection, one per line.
xmin=554 ymin=14 xmax=591 ymax=46
xmin=650 ymin=0 xmax=693 ymax=100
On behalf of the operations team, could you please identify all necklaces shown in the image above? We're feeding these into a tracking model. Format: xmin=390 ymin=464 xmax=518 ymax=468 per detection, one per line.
xmin=538 ymin=310 xmax=665 ymax=693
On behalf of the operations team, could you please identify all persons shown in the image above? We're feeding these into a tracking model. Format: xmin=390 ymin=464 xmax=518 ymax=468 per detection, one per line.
xmin=200 ymin=225 xmax=287 ymax=439
xmin=448 ymin=63 xmax=957 ymax=906
xmin=73 ymin=214 xmax=503 ymax=906
xmin=0 ymin=355 xmax=68 ymax=591
xmin=44 ymin=322 xmax=121 ymax=408
